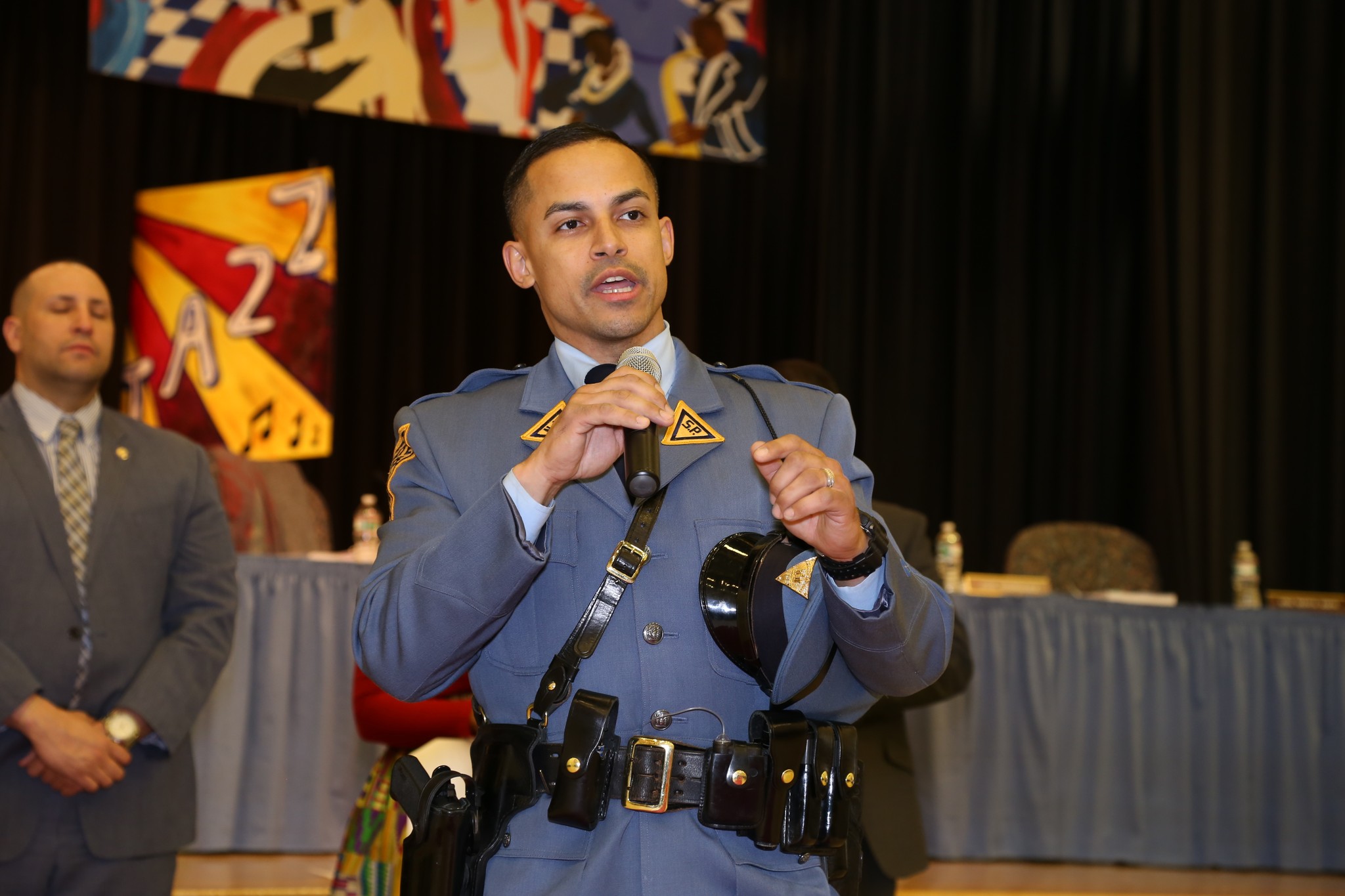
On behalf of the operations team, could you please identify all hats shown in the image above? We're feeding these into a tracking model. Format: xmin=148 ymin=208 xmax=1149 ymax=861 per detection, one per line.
xmin=701 ymin=510 xmax=879 ymax=725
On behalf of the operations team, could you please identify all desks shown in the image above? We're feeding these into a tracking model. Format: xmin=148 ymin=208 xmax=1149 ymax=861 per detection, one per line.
xmin=188 ymin=551 xmax=1345 ymax=878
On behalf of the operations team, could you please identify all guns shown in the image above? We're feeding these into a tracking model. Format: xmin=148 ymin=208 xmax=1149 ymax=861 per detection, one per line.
xmin=388 ymin=754 xmax=472 ymax=896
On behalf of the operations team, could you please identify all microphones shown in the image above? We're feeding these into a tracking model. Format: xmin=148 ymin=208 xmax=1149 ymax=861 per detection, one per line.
xmin=618 ymin=346 xmax=663 ymax=499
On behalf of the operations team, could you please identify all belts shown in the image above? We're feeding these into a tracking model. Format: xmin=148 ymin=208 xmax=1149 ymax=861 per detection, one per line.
xmin=533 ymin=738 xmax=706 ymax=812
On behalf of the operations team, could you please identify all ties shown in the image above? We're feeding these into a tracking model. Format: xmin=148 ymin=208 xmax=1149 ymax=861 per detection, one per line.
xmin=52 ymin=417 xmax=94 ymax=709
xmin=584 ymin=364 xmax=638 ymax=509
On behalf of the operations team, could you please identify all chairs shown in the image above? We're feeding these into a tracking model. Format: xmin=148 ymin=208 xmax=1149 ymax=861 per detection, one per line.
xmin=1006 ymin=520 xmax=1159 ymax=594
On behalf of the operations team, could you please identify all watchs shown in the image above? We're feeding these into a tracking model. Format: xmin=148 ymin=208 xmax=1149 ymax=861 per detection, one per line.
xmin=814 ymin=506 xmax=889 ymax=582
xmin=104 ymin=708 xmax=140 ymax=747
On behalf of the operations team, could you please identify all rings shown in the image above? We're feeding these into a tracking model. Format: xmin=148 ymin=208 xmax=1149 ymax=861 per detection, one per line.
xmin=821 ymin=467 xmax=834 ymax=488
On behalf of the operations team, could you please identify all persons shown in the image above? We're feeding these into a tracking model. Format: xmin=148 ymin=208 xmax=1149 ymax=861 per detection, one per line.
xmin=353 ymin=123 xmax=955 ymax=896
xmin=0 ymin=262 xmax=238 ymax=896
xmin=776 ymin=360 xmax=977 ymax=896
xmin=328 ymin=660 xmax=488 ymax=896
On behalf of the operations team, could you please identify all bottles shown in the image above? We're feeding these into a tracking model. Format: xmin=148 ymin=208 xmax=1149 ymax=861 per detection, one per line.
xmin=352 ymin=495 xmax=381 ymax=563
xmin=935 ymin=520 xmax=962 ymax=596
xmin=1233 ymin=542 xmax=1263 ymax=609
